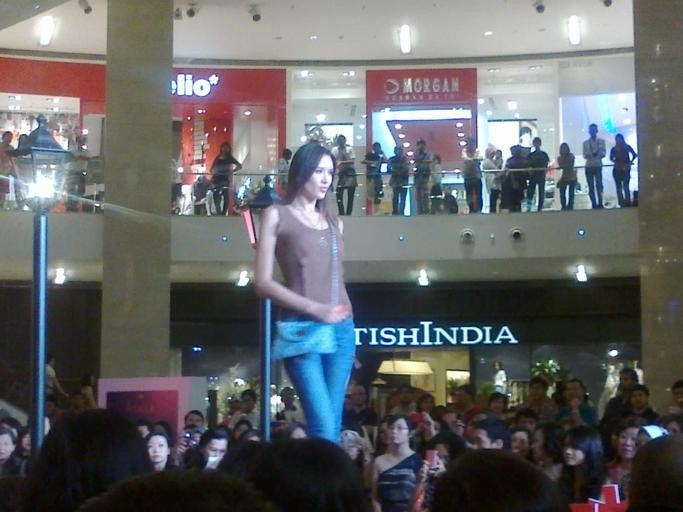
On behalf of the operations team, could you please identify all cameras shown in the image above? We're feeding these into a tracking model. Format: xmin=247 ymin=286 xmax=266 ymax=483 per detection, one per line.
xmin=185 ymin=432 xmax=196 ymax=448
xmin=407 ymin=412 xmax=424 ymax=424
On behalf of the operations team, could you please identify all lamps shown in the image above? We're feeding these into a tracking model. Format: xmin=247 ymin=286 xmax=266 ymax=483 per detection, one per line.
xmin=376 ymin=345 xmax=432 ymax=375
xmin=397 ymin=24 xmax=413 ymax=57
xmin=567 ymin=13 xmax=583 ymax=44
xmin=532 ymin=0 xmax=545 ymax=13
xmin=185 ymin=1 xmax=198 ymax=18
xmin=248 ymin=5 xmax=261 ymax=21
xmin=78 ymin=0 xmax=89 ymax=13
xmin=39 ymin=17 xmax=56 ymax=45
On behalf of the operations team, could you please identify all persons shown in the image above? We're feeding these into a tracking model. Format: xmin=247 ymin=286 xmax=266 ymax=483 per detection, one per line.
xmin=333 ymin=124 xmax=640 ymax=216
xmin=1 ymin=354 xmax=683 ymax=512
xmin=252 ymin=142 xmax=357 ymax=445
xmin=0 ymin=115 xmax=91 ymax=212
xmin=168 ymin=144 xmax=293 ymax=215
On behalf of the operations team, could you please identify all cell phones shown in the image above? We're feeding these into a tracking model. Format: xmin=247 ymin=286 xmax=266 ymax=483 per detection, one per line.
xmin=425 ymin=448 xmax=442 ymax=472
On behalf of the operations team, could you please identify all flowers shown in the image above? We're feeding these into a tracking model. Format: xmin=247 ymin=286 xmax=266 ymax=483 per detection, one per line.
xmin=531 ymin=359 xmax=562 ymax=378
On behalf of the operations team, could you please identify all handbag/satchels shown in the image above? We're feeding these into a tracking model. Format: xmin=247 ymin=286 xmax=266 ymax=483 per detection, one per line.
xmin=272 ymin=322 xmax=336 ymax=361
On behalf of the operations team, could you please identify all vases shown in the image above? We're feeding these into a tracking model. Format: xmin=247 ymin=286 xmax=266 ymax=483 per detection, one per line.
xmin=541 ymin=374 xmax=554 ymax=386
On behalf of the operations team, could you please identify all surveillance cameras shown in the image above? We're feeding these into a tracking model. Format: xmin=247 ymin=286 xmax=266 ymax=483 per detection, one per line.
xmin=78 ymin=0 xmax=92 ymax=15
xmin=459 ymin=228 xmax=473 ymax=242
xmin=186 ymin=7 xmax=198 ymax=19
xmin=533 ymin=1 xmax=546 ymax=14
xmin=510 ymin=226 xmax=525 ymax=242
xmin=604 ymin=0 xmax=613 ymax=8
xmin=251 ymin=11 xmax=264 ymax=25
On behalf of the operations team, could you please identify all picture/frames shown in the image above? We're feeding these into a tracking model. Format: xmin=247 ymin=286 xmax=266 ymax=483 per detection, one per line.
xmin=410 ymin=368 xmax=437 ymax=392
